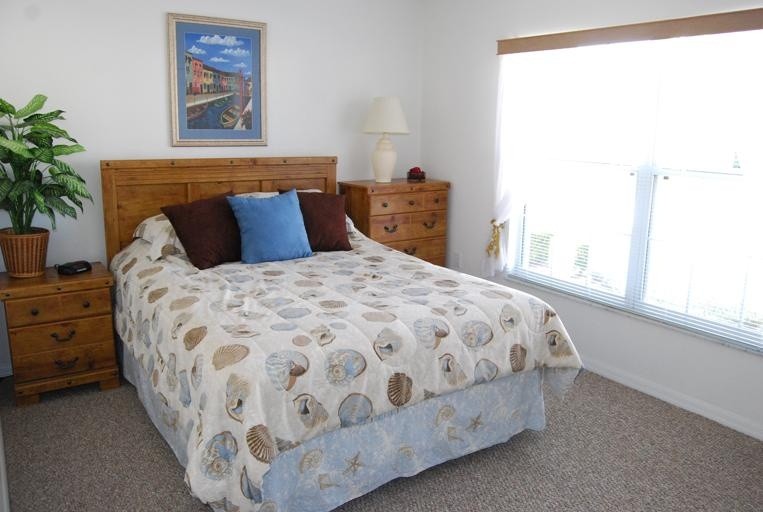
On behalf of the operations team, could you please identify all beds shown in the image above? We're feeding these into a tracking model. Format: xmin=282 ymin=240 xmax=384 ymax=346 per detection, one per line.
xmin=99 ymin=155 xmax=583 ymax=512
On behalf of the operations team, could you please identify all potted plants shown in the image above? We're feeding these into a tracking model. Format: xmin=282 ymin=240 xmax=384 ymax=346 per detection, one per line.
xmin=0 ymin=93 xmax=94 ymax=280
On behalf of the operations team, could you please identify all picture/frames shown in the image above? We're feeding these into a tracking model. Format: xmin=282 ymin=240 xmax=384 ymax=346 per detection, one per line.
xmin=166 ymin=12 xmax=269 ymax=147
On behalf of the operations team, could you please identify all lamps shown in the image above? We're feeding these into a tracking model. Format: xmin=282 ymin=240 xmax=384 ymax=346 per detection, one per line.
xmin=358 ymin=95 xmax=412 ymax=183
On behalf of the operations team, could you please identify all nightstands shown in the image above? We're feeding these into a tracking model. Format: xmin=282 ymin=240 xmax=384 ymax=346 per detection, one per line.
xmin=0 ymin=261 xmax=123 ymax=409
xmin=337 ymin=178 xmax=453 ymax=268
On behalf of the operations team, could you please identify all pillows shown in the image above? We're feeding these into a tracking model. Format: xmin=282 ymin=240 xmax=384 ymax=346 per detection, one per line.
xmin=225 ymin=190 xmax=313 ymax=263
xmin=276 ymin=187 xmax=352 ymax=252
xmin=132 ymin=213 xmax=185 ymax=262
xmin=160 ymin=189 xmax=242 ymax=270
